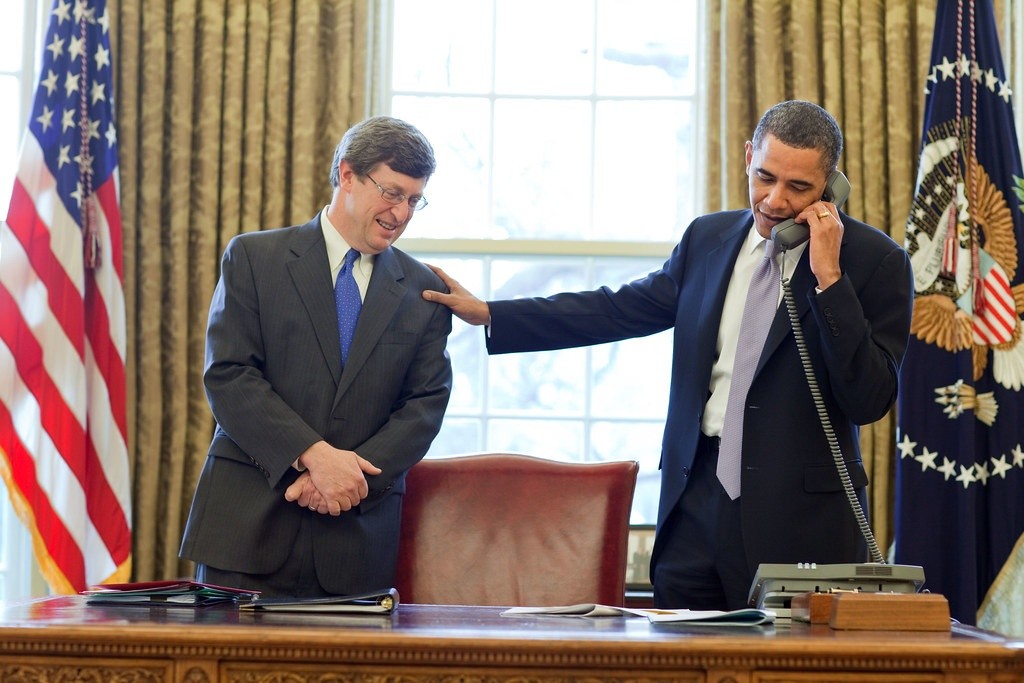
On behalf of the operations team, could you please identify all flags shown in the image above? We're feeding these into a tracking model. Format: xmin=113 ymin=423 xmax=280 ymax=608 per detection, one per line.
xmin=0 ymin=0 xmax=132 ymax=596
xmin=887 ymin=0 xmax=1024 ymax=645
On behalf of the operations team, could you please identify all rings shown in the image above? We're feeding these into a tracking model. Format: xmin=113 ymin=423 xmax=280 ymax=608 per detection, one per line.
xmin=817 ymin=212 xmax=829 ymax=218
xmin=309 ymin=504 xmax=318 ymax=510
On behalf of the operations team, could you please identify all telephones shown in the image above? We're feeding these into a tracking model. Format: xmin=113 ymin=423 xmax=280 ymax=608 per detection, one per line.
xmin=770 ymin=170 xmax=852 ymax=250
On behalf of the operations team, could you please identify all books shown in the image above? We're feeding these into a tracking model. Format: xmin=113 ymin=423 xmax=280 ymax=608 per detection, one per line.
xmin=78 ymin=580 xmax=261 ymax=607
xmin=238 ymin=588 xmax=400 ymax=616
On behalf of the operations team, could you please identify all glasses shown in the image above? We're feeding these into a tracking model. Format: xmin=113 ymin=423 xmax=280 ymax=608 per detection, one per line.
xmin=365 ymin=173 xmax=429 ymax=211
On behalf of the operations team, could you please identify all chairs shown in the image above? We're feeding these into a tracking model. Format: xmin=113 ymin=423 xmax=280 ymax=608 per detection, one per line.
xmin=398 ymin=453 xmax=638 ymax=607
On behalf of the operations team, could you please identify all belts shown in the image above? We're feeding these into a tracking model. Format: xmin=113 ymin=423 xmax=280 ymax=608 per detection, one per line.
xmin=703 ymin=438 xmax=720 ymax=463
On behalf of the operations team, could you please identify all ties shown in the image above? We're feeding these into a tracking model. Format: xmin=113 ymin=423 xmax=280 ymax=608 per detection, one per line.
xmin=333 ymin=249 xmax=362 ymax=368
xmin=716 ymin=239 xmax=787 ymax=501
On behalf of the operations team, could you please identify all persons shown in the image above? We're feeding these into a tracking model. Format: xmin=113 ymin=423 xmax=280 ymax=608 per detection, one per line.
xmin=422 ymin=100 xmax=913 ymax=612
xmin=178 ymin=117 xmax=453 ymax=598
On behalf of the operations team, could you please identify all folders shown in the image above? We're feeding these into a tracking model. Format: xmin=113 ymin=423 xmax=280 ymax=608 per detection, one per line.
xmin=238 ymin=586 xmax=404 ymax=617
xmin=78 ymin=579 xmax=261 ymax=606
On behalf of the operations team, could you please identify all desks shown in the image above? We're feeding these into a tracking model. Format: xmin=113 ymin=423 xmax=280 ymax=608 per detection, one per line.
xmin=1 ymin=597 xmax=1024 ymax=683
